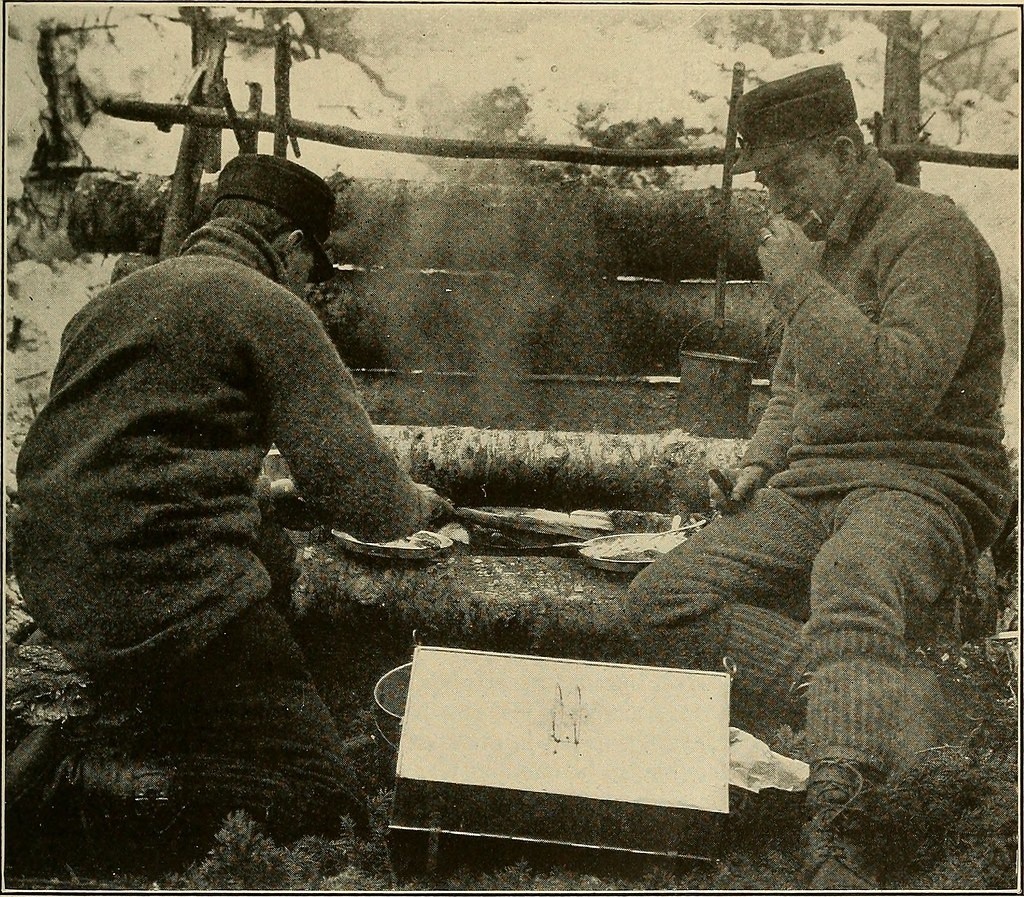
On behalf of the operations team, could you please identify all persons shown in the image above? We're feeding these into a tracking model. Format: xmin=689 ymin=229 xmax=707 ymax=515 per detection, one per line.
xmin=4 ymin=154 xmax=450 ymax=873
xmin=624 ymin=61 xmax=1012 ymax=889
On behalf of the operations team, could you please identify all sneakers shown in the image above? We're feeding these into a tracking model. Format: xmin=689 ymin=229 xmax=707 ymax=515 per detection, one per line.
xmin=5 ymin=725 xmax=64 ymax=808
xmin=799 ymin=759 xmax=883 ymax=889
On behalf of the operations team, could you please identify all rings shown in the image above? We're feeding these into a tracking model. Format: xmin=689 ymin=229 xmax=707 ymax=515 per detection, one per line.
xmin=762 ymin=232 xmax=775 ymax=243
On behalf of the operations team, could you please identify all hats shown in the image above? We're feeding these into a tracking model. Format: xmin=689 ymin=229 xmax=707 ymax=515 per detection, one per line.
xmin=725 ymin=63 xmax=859 ymax=176
xmin=209 ymin=154 xmax=337 ymax=285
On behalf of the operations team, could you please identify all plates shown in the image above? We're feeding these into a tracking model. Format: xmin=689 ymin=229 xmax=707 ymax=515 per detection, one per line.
xmin=580 ymin=535 xmax=689 ymax=573
xmin=332 ymin=529 xmax=452 ymax=561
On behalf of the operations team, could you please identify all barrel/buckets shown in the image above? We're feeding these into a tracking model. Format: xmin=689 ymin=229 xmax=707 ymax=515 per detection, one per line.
xmin=677 ymin=351 xmax=757 ymax=439
xmin=374 ymin=661 xmax=412 ymax=775
